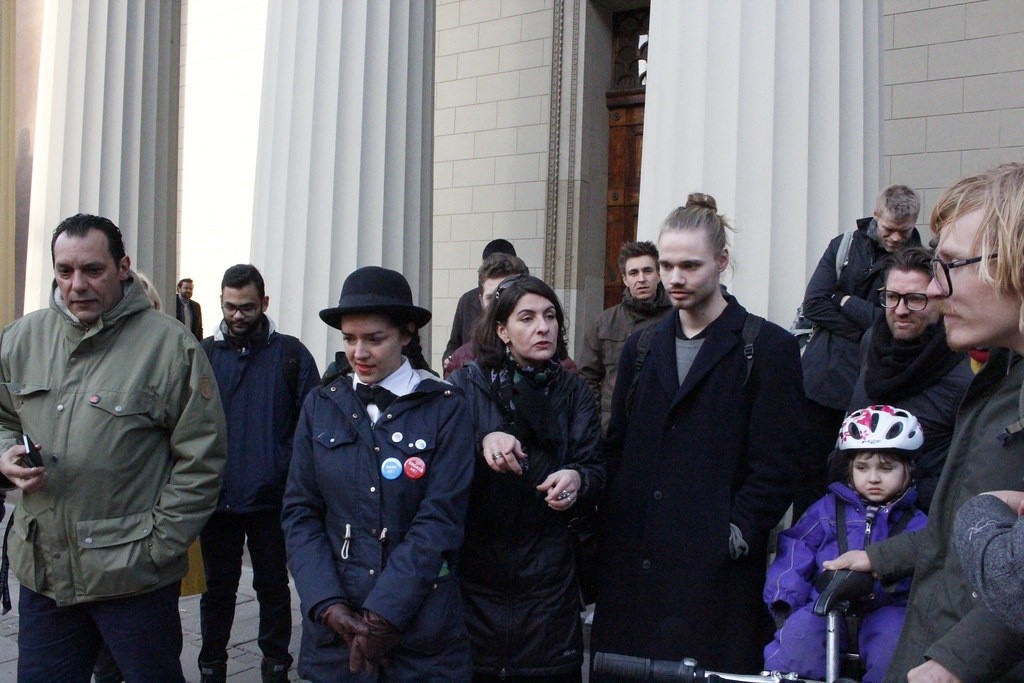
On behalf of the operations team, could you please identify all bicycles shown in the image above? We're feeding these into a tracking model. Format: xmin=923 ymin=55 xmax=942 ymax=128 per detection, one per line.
xmin=593 ymin=567 xmax=869 ymax=683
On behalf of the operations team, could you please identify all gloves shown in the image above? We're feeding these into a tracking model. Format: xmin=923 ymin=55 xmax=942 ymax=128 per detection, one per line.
xmin=321 ymin=603 xmax=401 ymax=673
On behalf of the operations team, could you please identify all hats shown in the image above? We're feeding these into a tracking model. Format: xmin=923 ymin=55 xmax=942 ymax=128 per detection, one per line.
xmin=319 ymin=268 xmax=432 ymax=330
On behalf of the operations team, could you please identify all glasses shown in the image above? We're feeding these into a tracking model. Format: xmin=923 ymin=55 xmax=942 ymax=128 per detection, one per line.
xmin=930 ymin=253 xmax=1000 ymax=298
xmin=494 ymin=273 xmax=523 ymax=310
xmin=876 ymin=287 xmax=932 ymax=311
xmin=220 ymin=298 xmax=260 ymax=317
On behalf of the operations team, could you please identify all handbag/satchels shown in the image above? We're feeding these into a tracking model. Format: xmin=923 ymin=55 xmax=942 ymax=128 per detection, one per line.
xmin=794 ymin=233 xmax=855 ymax=357
xmin=178 ymin=537 xmax=208 ymax=596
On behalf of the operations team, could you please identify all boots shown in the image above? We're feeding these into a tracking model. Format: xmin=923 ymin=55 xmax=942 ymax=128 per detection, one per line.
xmin=198 ymin=652 xmax=229 ymax=683
xmin=260 ymin=655 xmax=293 ymax=683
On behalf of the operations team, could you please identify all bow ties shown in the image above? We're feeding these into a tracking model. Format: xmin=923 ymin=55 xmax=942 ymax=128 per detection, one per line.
xmin=355 ymin=384 xmax=397 ymax=413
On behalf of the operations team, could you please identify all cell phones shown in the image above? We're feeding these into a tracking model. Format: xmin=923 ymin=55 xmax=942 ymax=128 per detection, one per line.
xmin=23 ymin=434 xmax=43 ymax=467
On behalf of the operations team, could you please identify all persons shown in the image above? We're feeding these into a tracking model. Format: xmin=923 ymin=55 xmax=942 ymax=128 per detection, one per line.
xmin=589 ymin=193 xmax=805 ymax=683
xmin=579 ymin=241 xmax=673 ymax=435
xmin=790 ymin=184 xmax=924 ymax=527
xmin=444 ymin=239 xmax=577 ymax=380
xmin=176 ymin=278 xmax=203 ymax=342
xmin=0 ymin=213 xmax=227 ymax=683
xmin=827 ymin=246 xmax=990 ymax=517
xmin=197 ymin=263 xmax=321 ymax=683
xmin=445 ymin=275 xmax=606 ymax=683
xmin=280 ymin=266 xmax=475 ymax=683
xmin=824 ymin=161 xmax=1024 ymax=683
xmin=952 ymin=490 xmax=1024 ymax=633
xmin=762 ymin=405 xmax=926 ymax=683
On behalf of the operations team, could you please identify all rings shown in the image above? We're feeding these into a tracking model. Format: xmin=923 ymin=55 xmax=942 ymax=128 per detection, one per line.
xmin=493 ymin=452 xmax=502 ymax=460
xmin=558 ymin=490 xmax=571 ymax=501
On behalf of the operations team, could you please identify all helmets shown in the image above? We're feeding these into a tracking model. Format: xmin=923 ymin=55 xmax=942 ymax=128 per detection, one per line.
xmin=836 ymin=404 xmax=925 ymax=451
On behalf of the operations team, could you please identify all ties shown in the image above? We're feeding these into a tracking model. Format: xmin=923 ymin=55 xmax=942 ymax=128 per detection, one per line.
xmin=183 ymin=304 xmax=190 ymax=329
